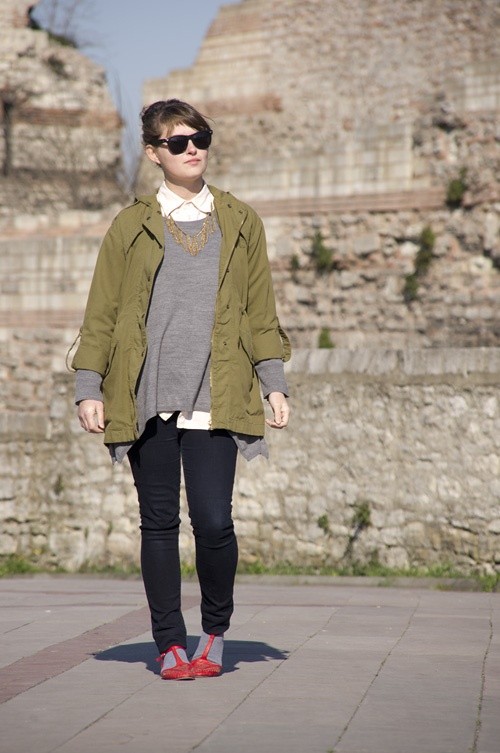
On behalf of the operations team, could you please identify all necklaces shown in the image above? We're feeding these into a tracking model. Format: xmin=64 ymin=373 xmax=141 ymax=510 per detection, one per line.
xmin=163 ymin=202 xmax=216 ymax=257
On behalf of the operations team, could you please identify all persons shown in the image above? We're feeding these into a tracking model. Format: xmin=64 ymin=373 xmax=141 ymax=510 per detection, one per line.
xmin=65 ymin=99 xmax=293 ymax=682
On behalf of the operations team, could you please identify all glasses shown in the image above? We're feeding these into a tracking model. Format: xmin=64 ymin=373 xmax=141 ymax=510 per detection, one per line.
xmin=152 ymin=129 xmax=215 ymax=156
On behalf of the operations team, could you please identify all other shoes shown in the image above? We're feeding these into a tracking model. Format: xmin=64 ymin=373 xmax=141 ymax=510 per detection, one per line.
xmin=188 ymin=631 xmax=226 ymax=675
xmin=155 ymin=643 xmax=193 ymax=679
